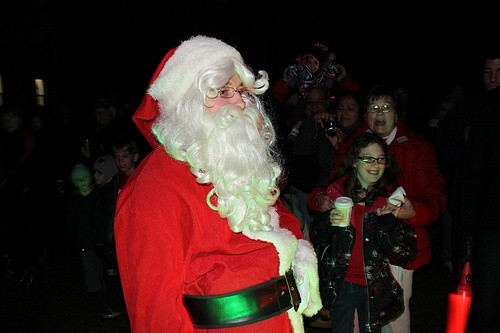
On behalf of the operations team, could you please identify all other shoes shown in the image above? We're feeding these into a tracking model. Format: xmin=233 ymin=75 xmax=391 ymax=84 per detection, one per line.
xmin=102 ymin=308 xmax=123 ymax=320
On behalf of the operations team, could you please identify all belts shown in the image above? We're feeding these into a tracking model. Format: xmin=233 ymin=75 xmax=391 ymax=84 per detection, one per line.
xmin=183 ymin=270 xmax=301 ymax=327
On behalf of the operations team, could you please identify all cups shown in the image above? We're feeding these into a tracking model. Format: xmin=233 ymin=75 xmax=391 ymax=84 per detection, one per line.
xmin=334 ymin=196 xmax=353 ymax=227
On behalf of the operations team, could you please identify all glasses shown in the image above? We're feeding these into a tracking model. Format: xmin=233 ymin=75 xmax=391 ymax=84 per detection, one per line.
xmin=355 ymin=155 xmax=389 ymax=165
xmin=213 ymin=87 xmax=251 ymax=100
xmin=365 ymin=103 xmax=394 ymax=113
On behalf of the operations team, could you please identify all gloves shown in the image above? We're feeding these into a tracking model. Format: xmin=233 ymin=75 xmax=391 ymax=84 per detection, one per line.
xmin=294 ymin=280 xmax=313 ymax=316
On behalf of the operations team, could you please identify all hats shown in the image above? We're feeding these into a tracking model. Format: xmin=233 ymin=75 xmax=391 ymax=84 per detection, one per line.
xmin=94 ymin=156 xmax=120 ymax=176
xmin=131 ymin=35 xmax=274 ymax=150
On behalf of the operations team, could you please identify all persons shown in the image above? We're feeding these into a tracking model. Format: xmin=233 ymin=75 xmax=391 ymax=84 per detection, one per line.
xmin=115 ymin=32 xmax=323 ymax=333
xmin=0 ymin=40 xmax=500 ymax=333
